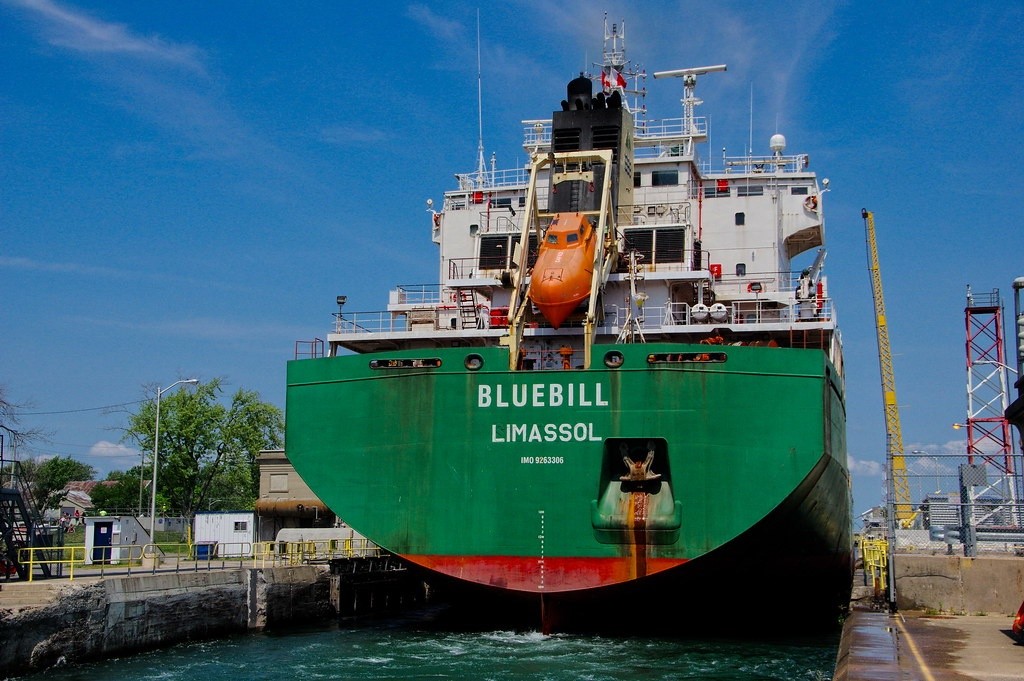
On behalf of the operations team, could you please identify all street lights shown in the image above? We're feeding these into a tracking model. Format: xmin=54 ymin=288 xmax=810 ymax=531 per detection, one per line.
xmin=906 ymin=469 xmax=922 ymax=504
xmin=150 ymin=379 xmax=200 ymax=552
xmin=912 ymin=450 xmax=940 ymax=493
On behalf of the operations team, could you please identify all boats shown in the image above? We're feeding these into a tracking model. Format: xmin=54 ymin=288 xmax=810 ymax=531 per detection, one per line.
xmin=283 ymin=7 xmax=854 ymax=634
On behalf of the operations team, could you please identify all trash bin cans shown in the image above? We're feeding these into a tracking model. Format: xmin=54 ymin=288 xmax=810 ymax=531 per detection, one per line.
xmin=192 ymin=540 xmax=219 ymax=560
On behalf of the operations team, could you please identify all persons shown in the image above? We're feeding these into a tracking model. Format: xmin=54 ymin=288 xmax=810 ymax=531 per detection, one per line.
xmin=100 ymin=509 xmax=107 ymax=516
xmin=60 ymin=510 xmax=79 ymax=532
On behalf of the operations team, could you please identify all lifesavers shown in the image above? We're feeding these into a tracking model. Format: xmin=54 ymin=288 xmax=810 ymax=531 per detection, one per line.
xmin=747 ymin=282 xmax=762 ymax=293
xmin=806 ymin=196 xmax=818 ymax=209
xmin=1012 ymin=600 xmax=1024 ymax=634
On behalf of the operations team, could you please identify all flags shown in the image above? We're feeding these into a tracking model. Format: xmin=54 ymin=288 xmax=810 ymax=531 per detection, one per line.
xmin=602 ymin=68 xmax=627 ymax=96
